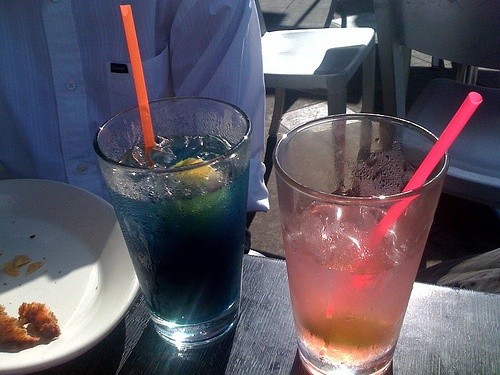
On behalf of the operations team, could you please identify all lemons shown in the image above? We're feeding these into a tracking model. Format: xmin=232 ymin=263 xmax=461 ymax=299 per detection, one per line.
xmin=167 ymin=158 xmax=218 ymax=185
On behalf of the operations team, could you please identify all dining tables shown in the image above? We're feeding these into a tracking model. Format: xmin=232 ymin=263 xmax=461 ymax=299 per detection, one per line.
xmin=41 ymin=252 xmax=500 ymax=375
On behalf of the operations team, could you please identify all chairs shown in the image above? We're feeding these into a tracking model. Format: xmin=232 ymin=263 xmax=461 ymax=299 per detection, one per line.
xmin=377 ymin=0 xmax=500 ymax=226
xmin=323 ymin=0 xmax=378 ymax=31
xmin=254 ymin=2 xmax=377 ymax=182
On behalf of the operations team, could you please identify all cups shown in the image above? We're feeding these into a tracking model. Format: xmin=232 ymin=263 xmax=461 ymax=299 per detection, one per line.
xmin=92 ymin=96 xmax=252 ymax=348
xmin=270 ymin=113 xmax=450 ymax=375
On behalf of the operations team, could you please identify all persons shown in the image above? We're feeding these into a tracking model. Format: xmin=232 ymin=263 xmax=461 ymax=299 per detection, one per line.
xmin=0 ymin=0 xmax=270 ymax=257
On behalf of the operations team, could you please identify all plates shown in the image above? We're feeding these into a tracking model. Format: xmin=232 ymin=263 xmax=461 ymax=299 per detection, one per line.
xmin=0 ymin=179 xmax=139 ymax=375
